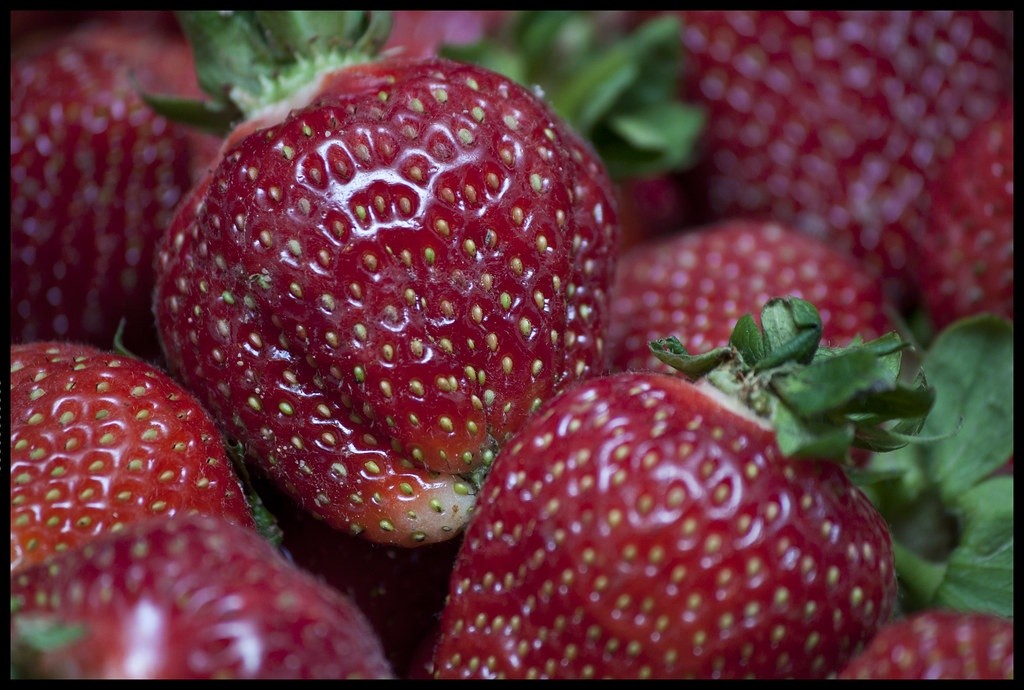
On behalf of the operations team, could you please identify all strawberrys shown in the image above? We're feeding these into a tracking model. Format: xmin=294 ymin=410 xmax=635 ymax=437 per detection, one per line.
xmin=9 ymin=10 xmax=1015 ymax=680
